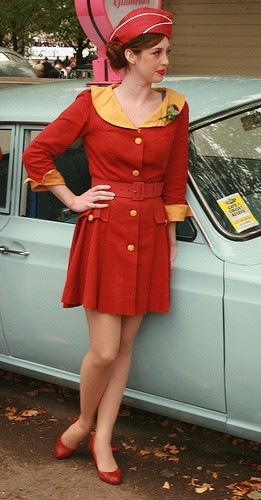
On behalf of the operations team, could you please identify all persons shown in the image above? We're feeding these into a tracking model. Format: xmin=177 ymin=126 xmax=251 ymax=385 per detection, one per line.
xmin=33 ymin=50 xmax=100 ymax=79
xmin=20 ymin=7 xmax=198 ymax=486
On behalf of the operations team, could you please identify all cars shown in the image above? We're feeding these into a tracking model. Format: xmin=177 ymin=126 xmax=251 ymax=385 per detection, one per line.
xmin=0 ymin=73 xmax=261 ymax=443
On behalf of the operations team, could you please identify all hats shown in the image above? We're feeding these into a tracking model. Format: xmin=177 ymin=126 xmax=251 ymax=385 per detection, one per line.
xmin=109 ymin=7 xmax=173 ymax=45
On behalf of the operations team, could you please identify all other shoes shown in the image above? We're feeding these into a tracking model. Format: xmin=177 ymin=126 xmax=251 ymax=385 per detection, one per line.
xmin=53 ymin=417 xmax=88 ymax=460
xmin=88 ymin=433 xmax=122 ymax=485
